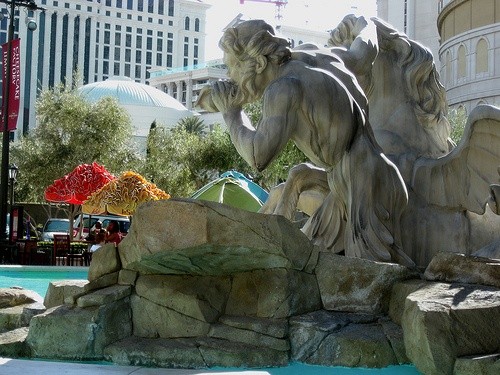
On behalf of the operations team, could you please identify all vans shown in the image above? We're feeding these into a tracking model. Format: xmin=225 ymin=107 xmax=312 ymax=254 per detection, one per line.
xmin=70 ymin=214 xmax=130 ymax=246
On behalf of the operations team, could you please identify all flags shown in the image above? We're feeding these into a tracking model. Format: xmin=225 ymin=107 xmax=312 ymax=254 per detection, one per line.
xmin=0 ymin=38 xmax=20 ymax=132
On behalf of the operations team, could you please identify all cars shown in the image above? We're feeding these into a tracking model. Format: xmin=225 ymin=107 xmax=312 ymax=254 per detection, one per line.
xmin=36 ymin=219 xmax=76 ymax=242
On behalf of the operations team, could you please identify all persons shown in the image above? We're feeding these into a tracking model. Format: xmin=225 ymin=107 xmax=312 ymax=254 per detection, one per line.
xmin=198 ymin=13 xmax=500 ymax=268
xmin=86 ymin=220 xmax=128 ymax=247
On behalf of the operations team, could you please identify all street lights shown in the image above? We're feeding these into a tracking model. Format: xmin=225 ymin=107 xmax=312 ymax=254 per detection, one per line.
xmin=8 ymin=162 xmax=19 ymax=250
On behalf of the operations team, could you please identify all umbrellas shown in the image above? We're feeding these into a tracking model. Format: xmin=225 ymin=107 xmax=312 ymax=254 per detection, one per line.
xmin=188 ymin=177 xmax=263 ymax=213
xmin=220 ymin=169 xmax=271 ymax=204
xmin=42 ymin=162 xmax=114 ymax=204
xmin=80 ymin=171 xmax=172 ymax=217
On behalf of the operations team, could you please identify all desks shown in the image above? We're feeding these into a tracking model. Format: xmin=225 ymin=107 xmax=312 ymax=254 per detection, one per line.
xmin=14 ymin=238 xmax=43 ymax=265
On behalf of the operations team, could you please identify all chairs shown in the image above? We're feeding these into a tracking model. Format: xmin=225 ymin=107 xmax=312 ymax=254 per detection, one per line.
xmin=16 ymin=239 xmax=38 ymax=265
xmin=53 ymin=235 xmax=84 ymax=266
